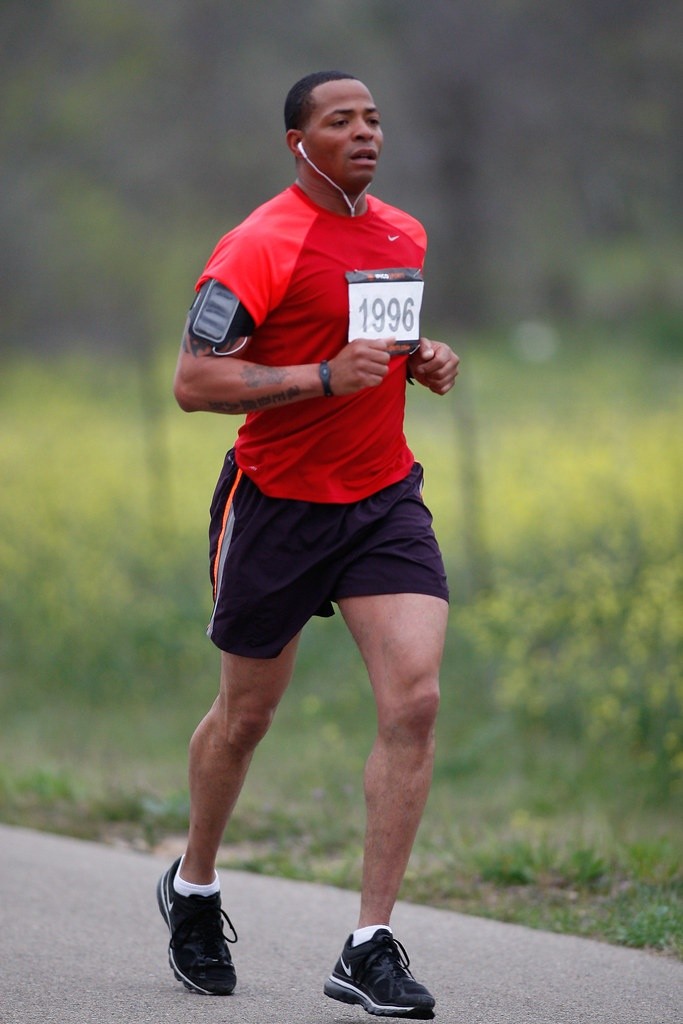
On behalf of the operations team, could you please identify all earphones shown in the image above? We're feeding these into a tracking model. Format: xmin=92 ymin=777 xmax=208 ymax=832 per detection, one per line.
xmin=297 ymin=142 xmax=308 ymax=159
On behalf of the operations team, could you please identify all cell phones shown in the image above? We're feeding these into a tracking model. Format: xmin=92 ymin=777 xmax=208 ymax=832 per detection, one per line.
xmin=188 ymin=278 xmax=240 ymax=348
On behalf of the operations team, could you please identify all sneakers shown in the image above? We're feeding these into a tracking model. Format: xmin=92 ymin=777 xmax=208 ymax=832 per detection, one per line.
xmin=156 ymin=856 xmax=238 ymax=995
xmin=324 ymin=928 xmax=435 ymax=1015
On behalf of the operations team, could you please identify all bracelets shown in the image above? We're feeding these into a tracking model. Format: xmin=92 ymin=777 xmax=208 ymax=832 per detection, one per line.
xmin=319 ymin=359 xmax=333 ymax=397
xmin=406 ymin=369 xmax=415 ymax=386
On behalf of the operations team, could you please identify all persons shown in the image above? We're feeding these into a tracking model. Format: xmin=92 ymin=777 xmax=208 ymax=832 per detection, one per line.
xmin=157 ymin=70 xmax=460 ymax=1021
